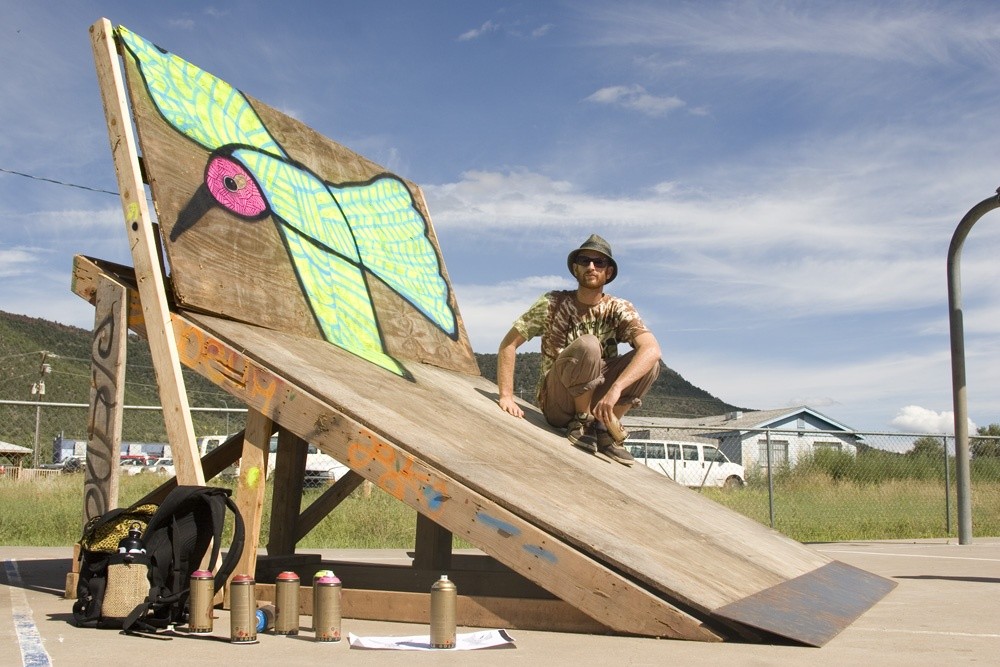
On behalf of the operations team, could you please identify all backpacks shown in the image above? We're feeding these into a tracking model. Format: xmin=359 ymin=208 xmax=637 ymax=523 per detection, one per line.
xmin=68 ymin=484 xmax=246 ymax=641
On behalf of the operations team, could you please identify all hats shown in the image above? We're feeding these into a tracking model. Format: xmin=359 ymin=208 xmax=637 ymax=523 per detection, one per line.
xmin=566 ymin=233 xmax=618 ymax=284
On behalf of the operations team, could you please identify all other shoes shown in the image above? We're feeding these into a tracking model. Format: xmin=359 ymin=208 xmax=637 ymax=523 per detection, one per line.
xmin=567 ymin=412 xmax=600 ymax=454
xmin=596 ymin=424 xmax=635 ymax=464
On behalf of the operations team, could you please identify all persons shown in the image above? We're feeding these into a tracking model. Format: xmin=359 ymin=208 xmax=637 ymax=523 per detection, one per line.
xmin=497 ymin=235 xmax=662 ymax=465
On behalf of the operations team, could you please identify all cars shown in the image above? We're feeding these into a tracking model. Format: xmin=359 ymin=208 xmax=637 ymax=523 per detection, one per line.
xmin=197 ymin=430 xmax=348 ymax=491
xmin=40 ymin=452 xmax=175 ymax=475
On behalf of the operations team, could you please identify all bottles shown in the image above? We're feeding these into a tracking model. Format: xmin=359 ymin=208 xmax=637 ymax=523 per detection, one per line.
xmin=116 ymin=522 xmax=146 ymax=554
xmin=312 ymin=570 xmax=334 ymax=631
xmin=230 ymin=575 xmax=257 ymax=644
xmin=430 ymin=575 xmax=457 ymax=649
xmin=316 ymin=571 xmax=342 ymax=642
xmin=189 ymin=570 xmax=214 ymax=632
xmin=275 ymin=572 xmax=300 ymax=634
xmin=606 ymin=413 xmax=630 ymax=444
xmin=254 ymin=604 xmax=277 ymax=632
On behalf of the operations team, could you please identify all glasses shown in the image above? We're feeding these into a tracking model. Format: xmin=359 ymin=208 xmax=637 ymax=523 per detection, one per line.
xmin=572 ymin=256 xmax=609 ymax=268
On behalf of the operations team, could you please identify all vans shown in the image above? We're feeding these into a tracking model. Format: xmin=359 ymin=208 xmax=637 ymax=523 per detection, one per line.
xmin=623 ymin=439 xmax=750 ymax=490
